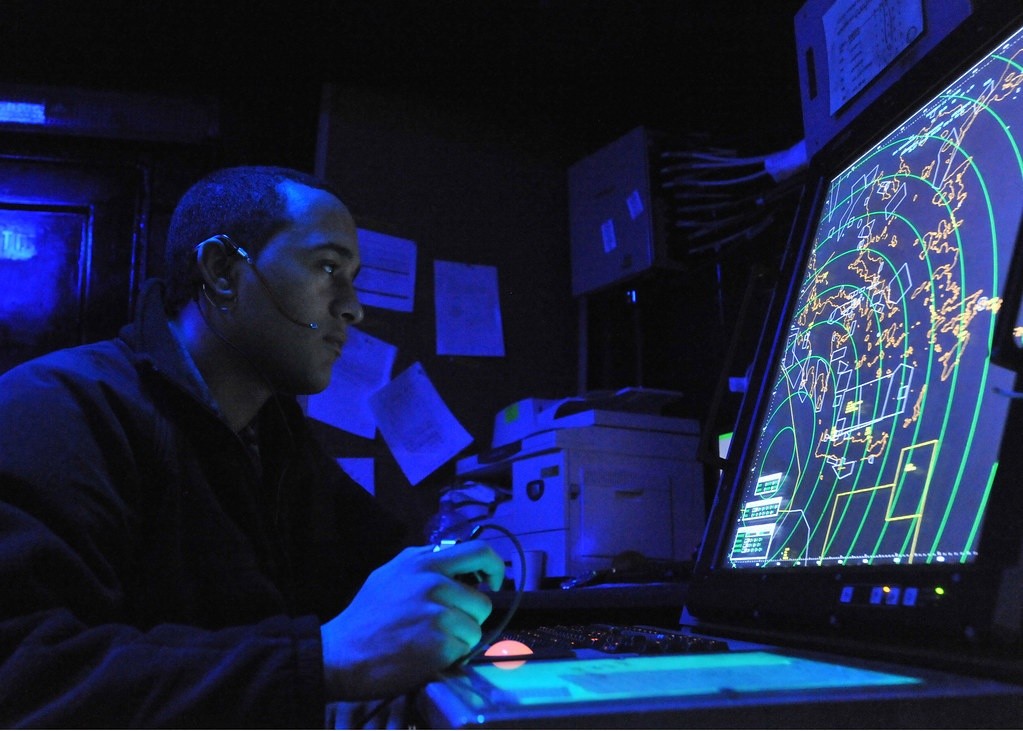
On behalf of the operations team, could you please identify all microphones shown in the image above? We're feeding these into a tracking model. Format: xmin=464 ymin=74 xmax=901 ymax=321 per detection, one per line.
xmin=210 ymin=235 xmax=318 ymax=330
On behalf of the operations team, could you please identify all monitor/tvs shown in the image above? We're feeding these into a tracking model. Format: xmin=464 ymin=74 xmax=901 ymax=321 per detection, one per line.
xmin=684 ymin=2 xmax=1023 ymax=632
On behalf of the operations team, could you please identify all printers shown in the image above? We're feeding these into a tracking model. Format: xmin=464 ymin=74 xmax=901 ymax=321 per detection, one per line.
xmin=436 ymin=386 xmax=708 ymax=591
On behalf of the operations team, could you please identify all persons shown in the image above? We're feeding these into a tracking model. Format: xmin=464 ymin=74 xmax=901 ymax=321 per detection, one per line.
xmin=0 ymin=166 xmax=505 ymax=730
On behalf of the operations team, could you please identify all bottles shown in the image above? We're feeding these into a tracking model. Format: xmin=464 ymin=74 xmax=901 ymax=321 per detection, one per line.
xmin=426 ymin=491 xmax=472 ymax=551
